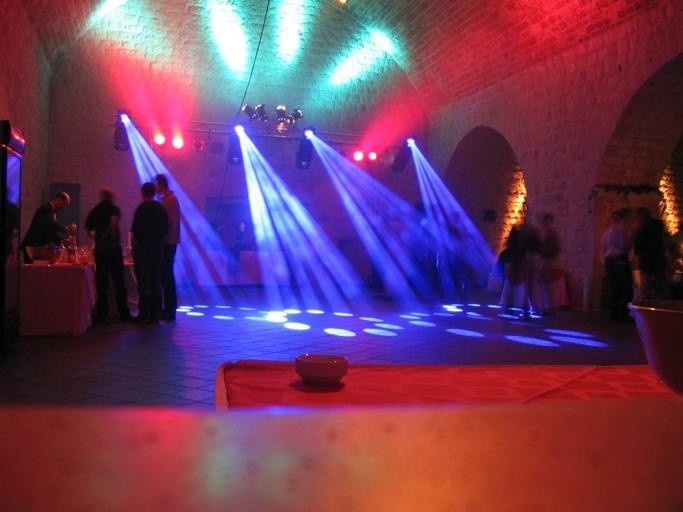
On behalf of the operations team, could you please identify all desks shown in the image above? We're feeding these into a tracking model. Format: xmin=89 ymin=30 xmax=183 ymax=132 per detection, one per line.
xmin=189 ymin=250 xmax=286 ymax=286
xmin=20 ymin=258 xmax=140 ymax=336
xmin=214 ymin=358 xmax=682 ymax=412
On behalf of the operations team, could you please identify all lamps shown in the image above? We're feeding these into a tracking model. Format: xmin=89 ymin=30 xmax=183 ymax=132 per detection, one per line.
xmin=113 ymin=111 xmax=131 ymax=151
xmin=228 ymin=125 xmax=242 ymax=165
xmin=296 ymin=127 xmax=315 ymax=169
xmin=240 ymin=102 xmax=303 ymax=127
xmin=392 ymin=137 xmax=415 ymax=172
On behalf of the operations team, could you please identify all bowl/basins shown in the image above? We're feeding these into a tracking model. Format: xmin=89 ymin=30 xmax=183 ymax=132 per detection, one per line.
xmin=26 ymin=246 xmax=59 ymax=264
xmin=295 ymin=353 xmax=349 ymax=385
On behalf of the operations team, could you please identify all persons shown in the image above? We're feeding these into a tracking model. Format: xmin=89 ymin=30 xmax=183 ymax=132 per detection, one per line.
xmin=500 ymin=207 xmax=677 ymax=321
xmin=0 ymin=173 xmax=180 ymax=326
xmin=215 ymin=213 xmax=251 ymax=274
